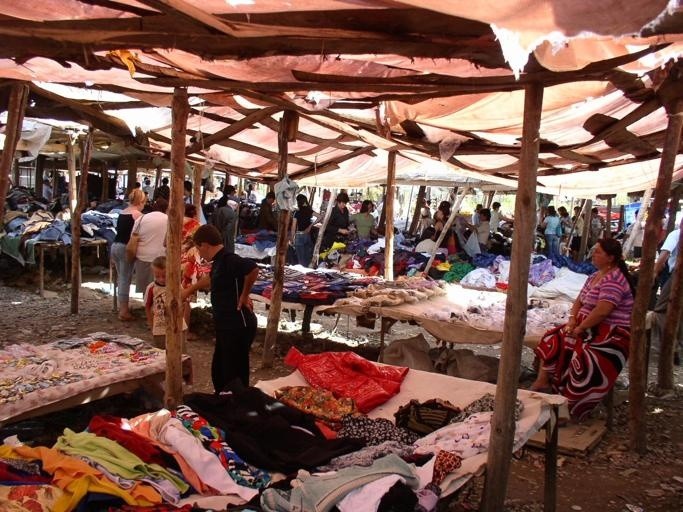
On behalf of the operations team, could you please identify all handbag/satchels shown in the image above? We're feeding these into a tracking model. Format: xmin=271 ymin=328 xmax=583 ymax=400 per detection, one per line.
xmin=556 ymin=225 xmax=562 ymax=237
xmin=125 ymin=232 xmax=140 ymax=263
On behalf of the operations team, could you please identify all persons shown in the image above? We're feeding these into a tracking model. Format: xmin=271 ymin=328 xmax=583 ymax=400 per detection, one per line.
xmin=178 ymin=224 xmax=258 ymax=395
xmin=627 ymin=209 xmax=645 ymax=260
xmin=539 ymin=206 xmax=607 ymax=261
xmin=257 ymin=190 xmax=380 ymax=253
xmin=110 ymin=177 xmax=256 ymax=351
xmin=529 ymin=237 xmax=635 ymax=424
xmin=414 ymin=201 xmax=513 ymax=257
xmin=42 ymin=175 xmax=53 ymax=205
xmin=654 ymin=228 xmax=681 ymax=313
